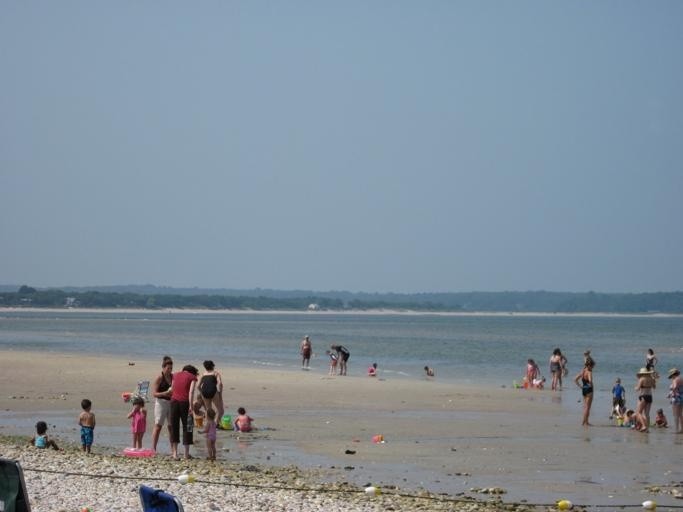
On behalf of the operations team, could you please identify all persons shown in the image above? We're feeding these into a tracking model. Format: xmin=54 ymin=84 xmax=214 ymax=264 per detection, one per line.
xmin=524 ymin=347 xmax=569 ymax=393
xmin=367 ymin=363 xmax=378 ymax=375
xmin=300 ymin=335 xmax=312 ymax=367
xmin=573 ymin=347 xmax=682 ymax=434
xmin=30 ymin=421 xmax=58 ymax=450
xmin=150 ymin=354 xmax=253 ymax=461
xmin=423 ymin=365 xmax=434 ymax=376
xmin=330 ymin=342 xmax=350 ymax=375
xmin=77 ymin=398 xmax=95 ymax=454
xmin=125 ymin=395 xmax=147 ymax=451
xmin=325 ymin=349 xmax=337 ymax=375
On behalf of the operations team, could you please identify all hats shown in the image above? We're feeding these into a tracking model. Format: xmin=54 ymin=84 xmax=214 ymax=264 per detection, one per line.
xmin=637 ymin=368 xmax=654 ymax=378
xmin=668 ymin=369 xmax=679 ymax=378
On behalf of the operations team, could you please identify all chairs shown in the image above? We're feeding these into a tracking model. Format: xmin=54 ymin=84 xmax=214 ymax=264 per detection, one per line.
xmin=122 ymin=379 xmax=149 ymax=404
